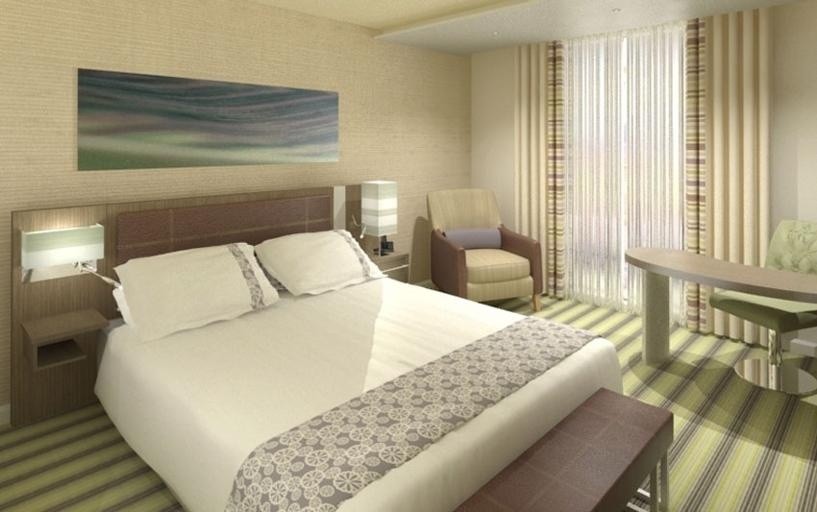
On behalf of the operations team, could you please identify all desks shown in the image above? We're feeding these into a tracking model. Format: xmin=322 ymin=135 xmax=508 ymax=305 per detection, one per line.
xmin=625 ymin=246 xmax=817 ymax=363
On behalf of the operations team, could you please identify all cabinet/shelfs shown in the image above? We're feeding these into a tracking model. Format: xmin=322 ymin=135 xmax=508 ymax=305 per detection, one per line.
xmin=20 ymin=307 xmax=108 ymax=371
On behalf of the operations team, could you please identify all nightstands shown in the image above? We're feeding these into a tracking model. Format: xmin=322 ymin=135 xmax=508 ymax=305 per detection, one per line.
xmin=365 ymin=249 xmax=410 ymax=283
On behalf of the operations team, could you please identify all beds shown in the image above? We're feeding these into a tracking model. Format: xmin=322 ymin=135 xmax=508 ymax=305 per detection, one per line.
xmin=97 ymin=195 xmax=622 ymax=511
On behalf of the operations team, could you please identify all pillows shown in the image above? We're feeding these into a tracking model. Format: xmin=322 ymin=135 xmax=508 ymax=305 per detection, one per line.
xmin=111 ymin=228 xmax=389 ymax=339
xmin=442 ymin=228 xmax=503 ymax=251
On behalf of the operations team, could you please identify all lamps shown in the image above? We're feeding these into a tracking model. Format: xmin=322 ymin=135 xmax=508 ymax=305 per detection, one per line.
xmin=361 ymin=181 xmax=399 ymax=256
xmin=21 ymin=223 xmax=106 ymax=268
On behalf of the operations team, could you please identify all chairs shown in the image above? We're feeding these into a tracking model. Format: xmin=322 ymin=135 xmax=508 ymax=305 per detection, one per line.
xmin=709 ymin=218 xmax=817 ymax=396
xmin=426 ymin=188 xmax=543 ymax=312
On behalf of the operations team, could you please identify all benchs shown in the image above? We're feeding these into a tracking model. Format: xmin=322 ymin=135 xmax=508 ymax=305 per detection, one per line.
xmin=459 ymin=388 xmax=672 ymax=510
